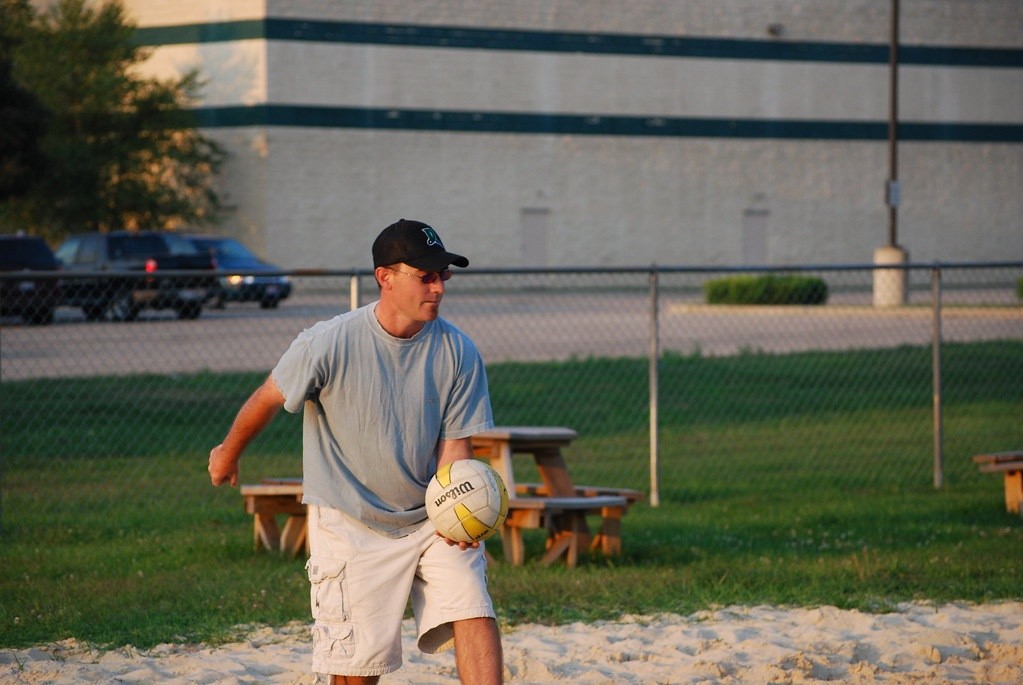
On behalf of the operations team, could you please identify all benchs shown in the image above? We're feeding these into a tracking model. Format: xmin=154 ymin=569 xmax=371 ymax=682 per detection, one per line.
xmin=237 ymin=476 xmax=646 ymax=572
xmin=974 ymin=450 xmax=1023 ymax=515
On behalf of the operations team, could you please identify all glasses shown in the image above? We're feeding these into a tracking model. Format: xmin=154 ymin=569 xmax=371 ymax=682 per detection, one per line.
xmin=384 ymin=267 xmax=452 ymax=284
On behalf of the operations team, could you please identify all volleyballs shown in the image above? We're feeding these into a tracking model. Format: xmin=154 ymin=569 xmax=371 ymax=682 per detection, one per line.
xmin=424 ymin=458 xmax=511 ymax=545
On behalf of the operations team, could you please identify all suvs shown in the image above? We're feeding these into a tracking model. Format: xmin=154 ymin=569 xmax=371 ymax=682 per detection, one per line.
xmin=1 ymin=234 xmax=64 ymax=326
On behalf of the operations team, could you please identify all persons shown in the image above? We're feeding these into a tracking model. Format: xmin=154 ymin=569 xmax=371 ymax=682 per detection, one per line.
xmin=208 ymin=218 xmax=503 ymax=685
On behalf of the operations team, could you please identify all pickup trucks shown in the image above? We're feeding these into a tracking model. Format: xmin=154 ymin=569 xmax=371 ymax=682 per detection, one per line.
xmin=53 ymin=232 xmax=218 ymax=321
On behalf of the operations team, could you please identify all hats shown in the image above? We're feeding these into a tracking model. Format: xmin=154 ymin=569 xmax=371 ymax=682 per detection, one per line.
xmin=372 ymin=219 xmax=469 ymax=270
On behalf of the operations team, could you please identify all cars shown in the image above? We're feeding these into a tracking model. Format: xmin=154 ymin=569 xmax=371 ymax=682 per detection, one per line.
xmin=190 ymin=237 xmax=295 ymax=313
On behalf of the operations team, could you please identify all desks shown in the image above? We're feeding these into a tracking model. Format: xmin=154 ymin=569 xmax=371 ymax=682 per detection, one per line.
xmin=280 ymin=428 xmax=592 ymax=563
